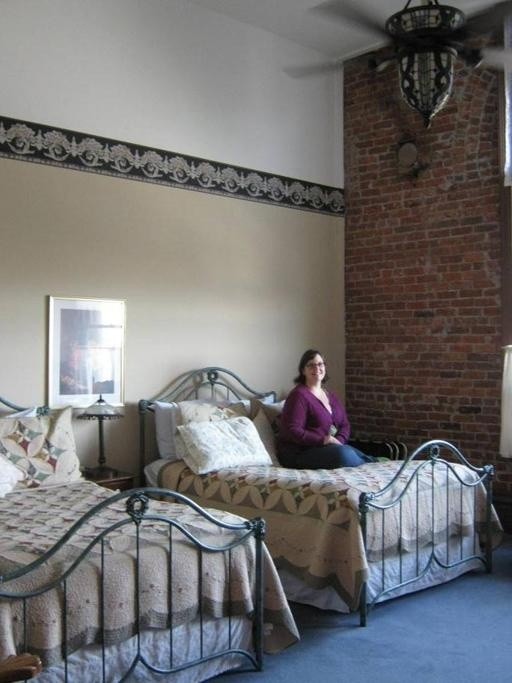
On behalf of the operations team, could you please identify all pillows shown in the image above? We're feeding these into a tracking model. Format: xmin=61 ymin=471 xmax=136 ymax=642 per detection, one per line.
xmin=154 ymin=392 xmax=288 ymax=475
xmin=0 ymin=404 xmax=86 ymax=496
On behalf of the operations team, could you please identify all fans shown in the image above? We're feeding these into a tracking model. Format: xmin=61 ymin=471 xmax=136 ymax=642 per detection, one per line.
xmin=278 ymin=0 xmax=510 ymax=76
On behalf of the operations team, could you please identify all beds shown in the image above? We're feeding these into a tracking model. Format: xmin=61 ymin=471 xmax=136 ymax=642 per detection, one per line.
xmin=139 ymin=366 xmax=503 ymax=626
xmin=0 ymin=397 xmax=299 ymax=682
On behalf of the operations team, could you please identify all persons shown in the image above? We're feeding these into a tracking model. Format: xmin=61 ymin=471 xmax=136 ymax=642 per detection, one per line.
xmin=278 ymin=349 xmax=390 ymax=469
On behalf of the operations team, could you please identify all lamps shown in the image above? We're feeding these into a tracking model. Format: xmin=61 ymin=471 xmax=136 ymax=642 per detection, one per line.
xmin=382 ymin=1 xmax=470 ymax=135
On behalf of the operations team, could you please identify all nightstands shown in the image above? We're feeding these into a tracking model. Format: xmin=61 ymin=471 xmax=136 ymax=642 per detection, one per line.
xmin=81 ymin=464 xmax=140 ymax=492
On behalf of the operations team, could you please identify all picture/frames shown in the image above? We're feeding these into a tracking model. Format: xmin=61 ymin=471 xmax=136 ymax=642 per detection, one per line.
xmin=46 ymin=293 xmax=130 ymax=411
